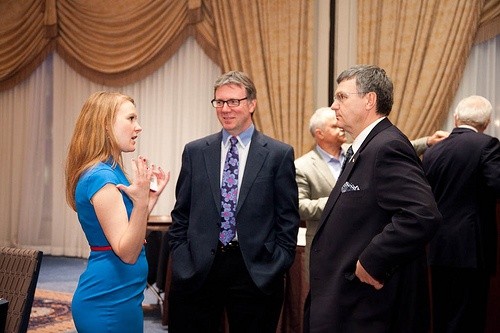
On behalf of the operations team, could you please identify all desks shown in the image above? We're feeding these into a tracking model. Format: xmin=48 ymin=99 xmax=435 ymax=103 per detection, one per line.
xmin=147 ymin=215 xmax=307 ymax=333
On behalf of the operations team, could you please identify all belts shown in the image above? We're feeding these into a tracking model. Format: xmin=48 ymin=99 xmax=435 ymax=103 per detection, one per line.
xmin=216 ymin=241 xmax=240 ymax=252
xmin=90 ymin=240 xmax=147 ymax=252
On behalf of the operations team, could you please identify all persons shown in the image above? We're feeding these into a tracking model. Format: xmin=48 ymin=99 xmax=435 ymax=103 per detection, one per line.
xmin=422 ymin=94 xmax=500 ymax=333
xmin=292 ymin=107 xmax=454 ymax=333
xmin=166 ymin=71 xmax=300 ymax=333
xmin=65 ymin=91 xmax=170 ymax=333
xmin=303 ymin=64 xmax=442 ymax=333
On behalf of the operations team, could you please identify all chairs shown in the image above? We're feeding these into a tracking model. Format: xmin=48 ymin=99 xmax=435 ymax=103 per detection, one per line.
xmin=0 ymin=246 xmax=43 ymax=333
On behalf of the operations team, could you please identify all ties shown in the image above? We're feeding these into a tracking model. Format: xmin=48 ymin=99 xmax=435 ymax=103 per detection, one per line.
xmin=339 ymin=145 xmax=354 ymax=179
xmin=219 ymin=137 xmax=239 ymax=246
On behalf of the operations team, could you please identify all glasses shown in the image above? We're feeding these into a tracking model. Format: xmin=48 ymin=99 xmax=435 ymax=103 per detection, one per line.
xmin=211 ymin=97 xmax=248 ymax=107
xmin=334 ymin=92 xmax=370 ymax=102
